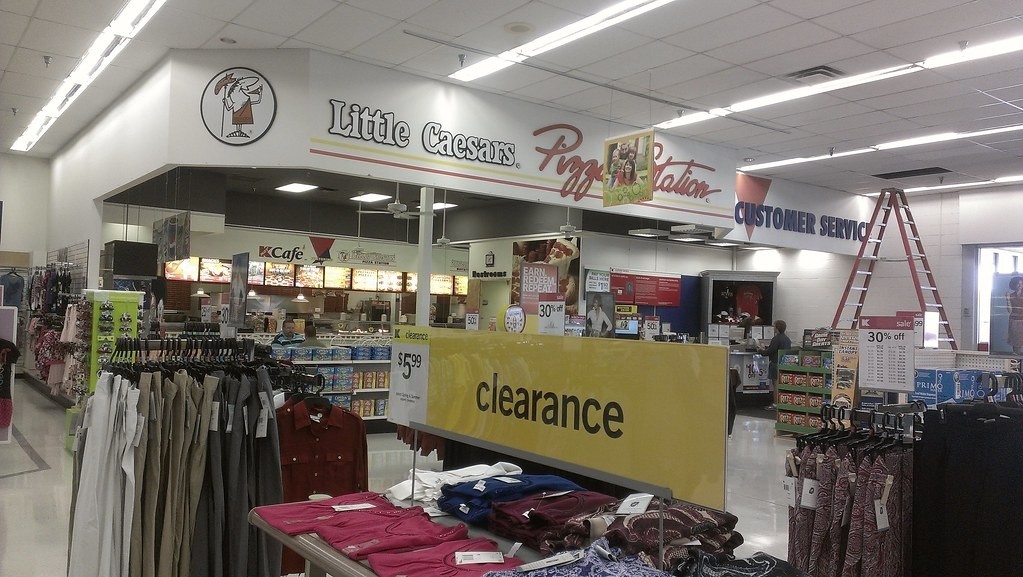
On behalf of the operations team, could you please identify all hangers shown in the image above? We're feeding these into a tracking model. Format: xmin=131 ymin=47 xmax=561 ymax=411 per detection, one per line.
xmin=7 ymin=267 xmax=20 ymax=277
xmin=796 ymin=400 xmax=927 ymax=463
xmin=104 ymin=336 xmax=332 ymax=411
xmin=27 ymin=262 xmax=71 ymax=276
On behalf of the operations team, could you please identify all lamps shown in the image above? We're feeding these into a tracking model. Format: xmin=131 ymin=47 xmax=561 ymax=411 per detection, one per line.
xmin=629 ymin=224 xmax=741 ymax=247
xmin=190 ymin=288 xmax=210 ymax=299
xmin=246 ymin=284 xmax=261 ymax=300
xmin=290 ymin=287 xmax=308 ymax=303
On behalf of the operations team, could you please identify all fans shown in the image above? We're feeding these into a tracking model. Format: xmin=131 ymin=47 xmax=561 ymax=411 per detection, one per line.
xmin=411 ymin=189 xmax=471 ymax=251
xmin=357 ymin=182 xmax=437 ymax=219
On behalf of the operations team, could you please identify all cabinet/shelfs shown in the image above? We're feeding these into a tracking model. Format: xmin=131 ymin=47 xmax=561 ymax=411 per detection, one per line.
xmin=703 ymin=268 xmax=779 ymax=395
xmin=297 ymin=357 xmax=393 ymax=418
xmin=773 ymin=349 xmax=830 ymax=437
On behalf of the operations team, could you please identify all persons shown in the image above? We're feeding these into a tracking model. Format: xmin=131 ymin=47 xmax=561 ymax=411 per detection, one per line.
xmin=1006 ymin=277 xmax=1023 ymax=355
xmin=271 ymin=319 xmax=305 ymax=346
xmin=586 ymin=294 xmax=613 ymax=338
xmin=299 ymin=326 xmax=327 ymax=348
xmin=607 ymin=143 xmax=643 ymax=189
xmin=753 ymin=320 xmax=792 ymax=412
xmin=231 ymin=258 xmax=245 ymax=319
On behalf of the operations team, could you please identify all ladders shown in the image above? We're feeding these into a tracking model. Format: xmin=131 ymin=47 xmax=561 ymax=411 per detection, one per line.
xmin=830 ymin=186 xmax=960 ymax=350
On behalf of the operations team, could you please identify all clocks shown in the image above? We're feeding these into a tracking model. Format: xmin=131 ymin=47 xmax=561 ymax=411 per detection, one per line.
xmin=486 ymin=253 xmax=495 ymax=266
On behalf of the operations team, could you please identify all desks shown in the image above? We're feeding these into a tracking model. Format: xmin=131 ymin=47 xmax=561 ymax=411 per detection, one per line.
xmin=250 ymin=495 xmax=544 ymax=577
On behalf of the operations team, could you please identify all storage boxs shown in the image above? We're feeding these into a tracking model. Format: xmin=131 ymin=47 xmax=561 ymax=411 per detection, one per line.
xmin=273 ymin=344 xmax=392 ymax=416
xmin=911 ymin=367 xmax=1007 ymax=410
